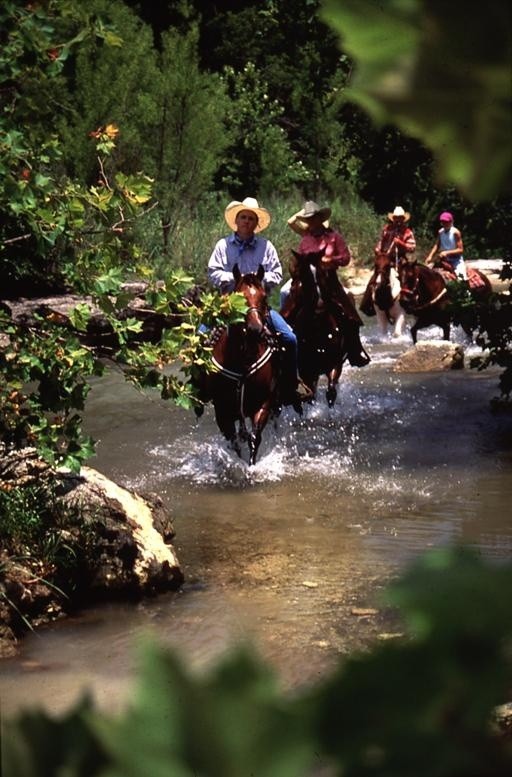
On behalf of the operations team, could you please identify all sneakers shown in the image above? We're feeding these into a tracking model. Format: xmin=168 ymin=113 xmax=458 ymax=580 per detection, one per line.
xmin=296 ymin=383 xmax=307 ymax=396
xmin=348 ymin=354 xmax=369 ymax=367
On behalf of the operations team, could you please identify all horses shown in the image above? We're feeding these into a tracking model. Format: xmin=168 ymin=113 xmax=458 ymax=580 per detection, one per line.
xmin=394 ymin=254 xmax=493 ymax=345
xmin=209 ymin=262 xmax=282 ymax=469
xmin=277 ymin=242 xmax=350 ymax=412
xmin=371 ymin=244 xmax=413 ymax=338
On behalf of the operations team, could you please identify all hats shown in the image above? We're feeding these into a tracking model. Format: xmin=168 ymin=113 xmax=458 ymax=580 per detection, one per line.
xmin=387 ymin=206 xmax=411 ymax=223
xmin=224 ymin=197 xmax=271 ymax=234
xmin=296 ymin=200 xmax=332 ymax=222
xmin=439 ymin=212 xmax=454 ymax=222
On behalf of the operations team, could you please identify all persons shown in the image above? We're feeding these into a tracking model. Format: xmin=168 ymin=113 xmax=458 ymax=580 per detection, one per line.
xmin=198 ymin=197 xmax=308 ymax=399
xmin=279 ymin=200 xmax=370 ymax=367
xmin=425 ymin=211 xmax=467 ymax=281
xmin=374 ymin=205 xmax=417 ymax=269
